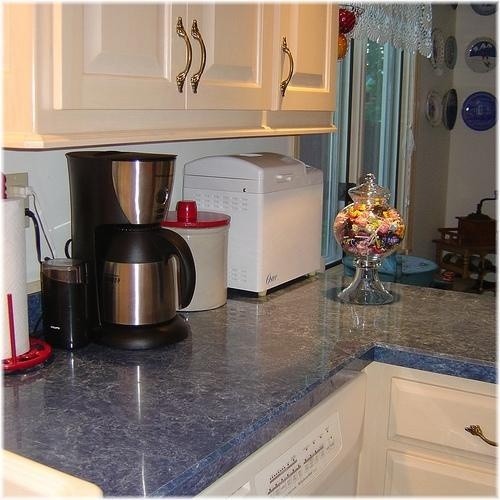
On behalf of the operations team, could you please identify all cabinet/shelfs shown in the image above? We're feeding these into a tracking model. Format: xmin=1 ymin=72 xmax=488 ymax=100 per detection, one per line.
xmin=380 ymin=377 xmax=496 ymax=496
xmin=276 ymin=3 xmax=340 ymax=136
xmin=2 ymin=3 xmax=275 ymax=151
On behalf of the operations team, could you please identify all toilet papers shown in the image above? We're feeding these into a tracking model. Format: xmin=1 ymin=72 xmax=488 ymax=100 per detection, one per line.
xmin=2 ymin=195 xmax=30 ymax=362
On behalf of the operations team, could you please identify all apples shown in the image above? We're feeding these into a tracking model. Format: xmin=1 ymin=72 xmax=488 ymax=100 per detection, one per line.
xmin=340 ymin=9 xmax=356 ymax=33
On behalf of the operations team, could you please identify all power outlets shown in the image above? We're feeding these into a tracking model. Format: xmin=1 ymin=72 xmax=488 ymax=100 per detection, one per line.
xmin=4 ymin=171 xmax=31 ymax=228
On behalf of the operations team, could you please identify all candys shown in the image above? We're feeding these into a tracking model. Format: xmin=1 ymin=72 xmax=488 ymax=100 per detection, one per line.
xmin=334 ymin=197 xmax=406 ymax=256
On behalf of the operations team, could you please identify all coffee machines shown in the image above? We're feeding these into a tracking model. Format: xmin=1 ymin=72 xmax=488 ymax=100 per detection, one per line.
xmin=64 ymin=151 xmax=195 ymax=345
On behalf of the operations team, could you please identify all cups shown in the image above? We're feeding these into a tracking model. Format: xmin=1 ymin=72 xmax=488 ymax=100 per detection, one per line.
xmin=38 ymin=258 xmax=90 ymax=346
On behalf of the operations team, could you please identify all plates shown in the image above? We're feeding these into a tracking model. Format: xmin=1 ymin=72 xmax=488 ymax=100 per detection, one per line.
xmin=469 ymin=5 xmax=495 ymax=16
xmin=445 ymin=36 xmax=459 ymax=69
xmin=462 ymin=91 xmax=496 ymax=131
xmin=464 ymin=36 xmax=496 ymax=73
xmin=442 ymin=89 xmax=459 ymax=129
xmin=424 ymin=88 xmax=444 ymax=127
xmin=430 ymin=28 xmax=445 ymax=68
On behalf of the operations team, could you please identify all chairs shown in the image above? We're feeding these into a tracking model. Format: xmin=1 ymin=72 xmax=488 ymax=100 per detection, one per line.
xmin=432 ymin=226 xmax=496 ymax=291
